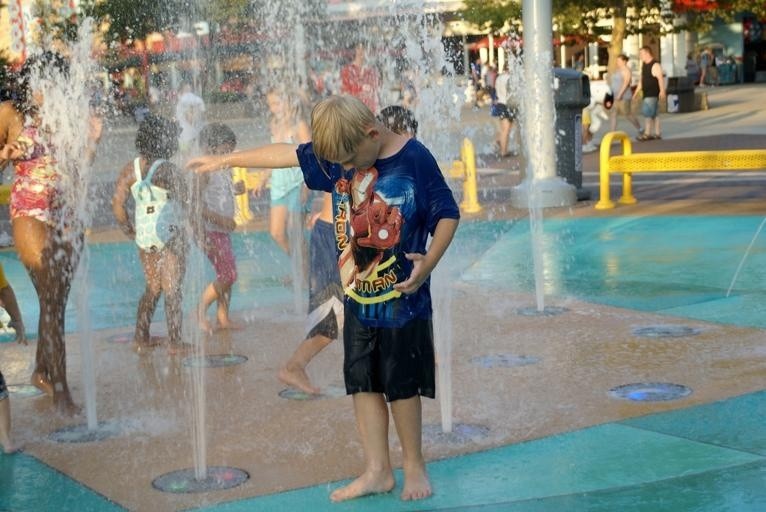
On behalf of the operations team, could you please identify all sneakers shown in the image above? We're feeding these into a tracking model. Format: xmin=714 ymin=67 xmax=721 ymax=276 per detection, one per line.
xmin=582 ymin=140 xmax=597 ymax=153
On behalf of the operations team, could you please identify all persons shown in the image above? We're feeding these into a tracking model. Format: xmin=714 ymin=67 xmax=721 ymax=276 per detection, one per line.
xmin=3 ymin=273 xmax=29 ymax=454
xmin=177 ymin=84 xmax=207 ymax=152
xmin=632 ymin=46 xmax=665 ymax=142
xmin=188 ymin=122 xmax=246 ymax=331
xmin=278 ymin=106 xmax=418 ymax=394
xmin=186 ymin=94 xmax=461 ymax=501
xmin=112 ymin=114 xmax=196 ymax=355
xmin=307 ymin=38 xmax=421 ymax=116
xmin=582 ymin=94 xmax=607 ymax=152
xmin=88 ymin=69 xmax=253 ymax=123
xmin=471 ymin=58 xmax=523 ymax=159
xmin=2 ymin=51 xmax=102 ymax=419
xmin=685 ymin=46 xmax=717 ymax=88
xmin=572 ymin=53 xmax=584 ymax=72
xmin=252 ymin=82 xmax=313 ymax=291
xmin=610 ymin=54 xmax=644 ymax=135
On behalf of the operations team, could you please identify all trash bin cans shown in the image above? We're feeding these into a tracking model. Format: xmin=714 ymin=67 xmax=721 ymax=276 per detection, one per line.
xmin=553 ymin=68 xmax=591 ymax=202
xmin=666 ymin=77 xmax=695 ymax=113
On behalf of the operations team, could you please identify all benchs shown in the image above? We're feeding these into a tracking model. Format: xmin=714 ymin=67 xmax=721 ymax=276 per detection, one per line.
xmin=595 ymin=130 xmax=766 ymax=212
xmin=233 ymin=138 xmax=481 ymax=223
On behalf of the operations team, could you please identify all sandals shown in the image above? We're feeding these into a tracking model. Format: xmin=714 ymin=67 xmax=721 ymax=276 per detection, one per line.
xmin=636 ymin=131 xmax=661 ymax=140
